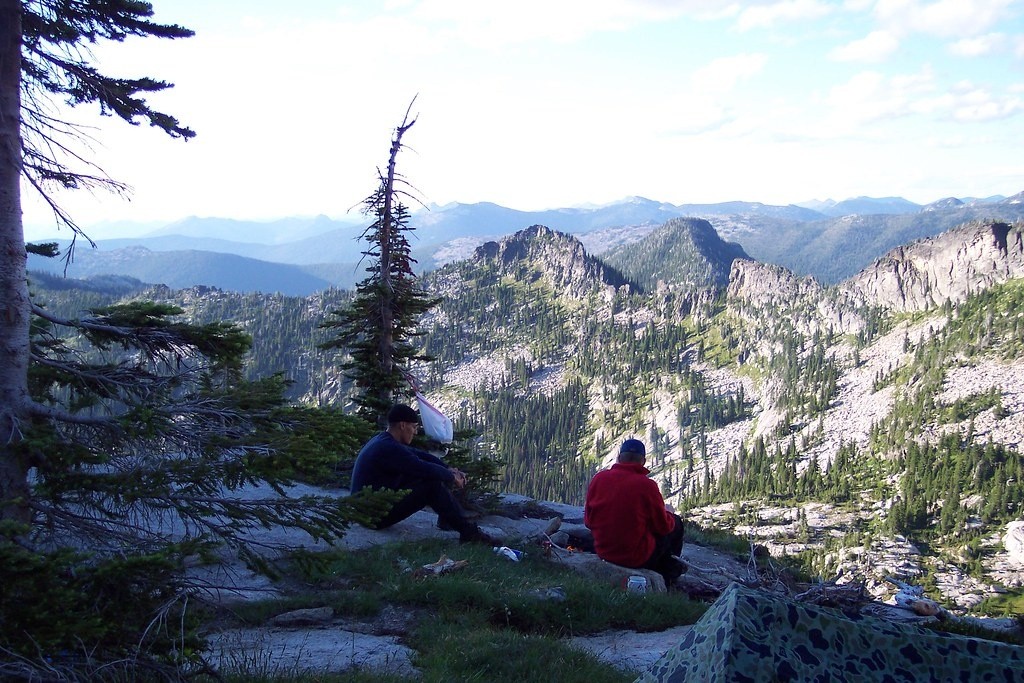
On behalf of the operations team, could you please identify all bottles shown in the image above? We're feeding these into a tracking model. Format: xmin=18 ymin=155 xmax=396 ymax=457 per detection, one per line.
xmin=493 ymin=546 xmax=528 ymax=561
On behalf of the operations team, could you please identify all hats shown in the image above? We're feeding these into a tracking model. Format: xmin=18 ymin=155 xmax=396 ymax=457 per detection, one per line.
xmin=387 ymin=404 xmax=420 ymax=424
xmin=620 ymin=439 xmax=646 ymax=456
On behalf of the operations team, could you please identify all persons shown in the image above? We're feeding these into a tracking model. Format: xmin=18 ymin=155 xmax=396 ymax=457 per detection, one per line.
xmin=349 ymin=404 xmax=503 ymax=548
xmin=584 ymin=439 xmax=684 ymax=583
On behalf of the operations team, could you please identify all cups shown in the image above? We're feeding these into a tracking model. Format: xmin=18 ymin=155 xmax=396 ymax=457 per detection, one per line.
xmin=626 ymin=576 xmax=646 ymax=598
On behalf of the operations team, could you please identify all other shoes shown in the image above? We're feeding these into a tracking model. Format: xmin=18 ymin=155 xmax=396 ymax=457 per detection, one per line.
xmin=437 ymin=516 xmax=468 ymax=531
xmin=681 ymin=554 xmax=690 ymax=573
xmin=458 ymin=523 xmax=503 ymax=547
